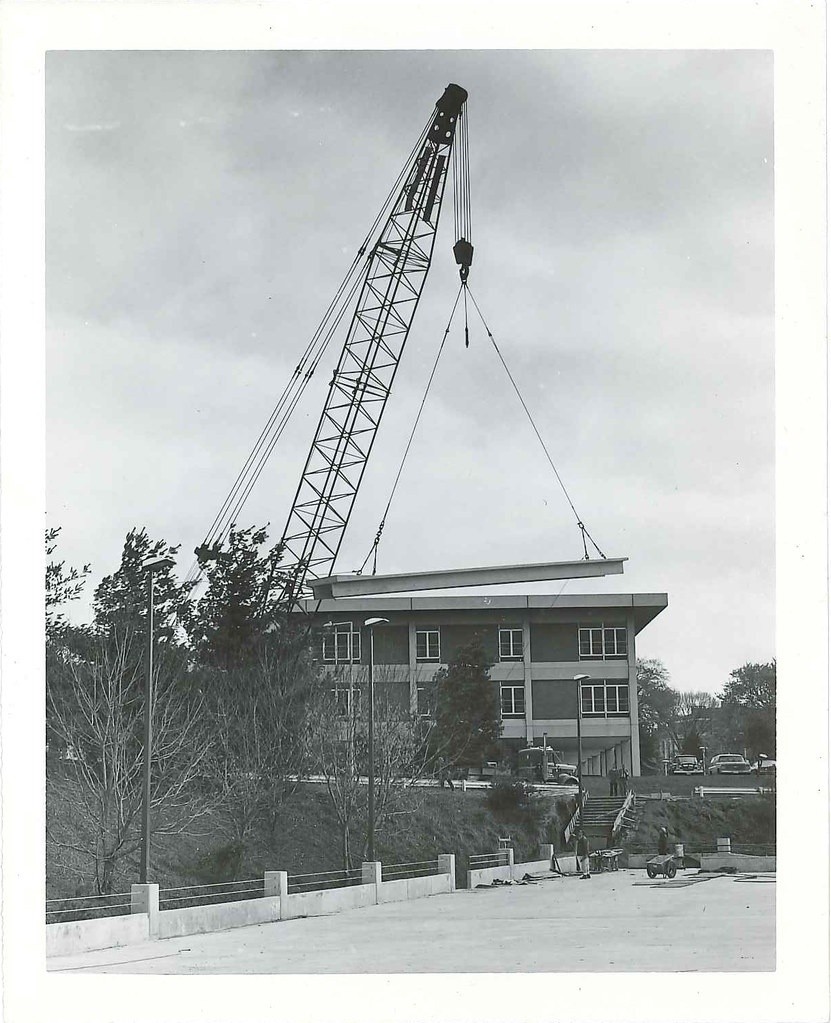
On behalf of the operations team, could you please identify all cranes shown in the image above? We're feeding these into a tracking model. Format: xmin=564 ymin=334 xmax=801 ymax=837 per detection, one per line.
xmin=214 ymin=83 xmax=474 ymax=650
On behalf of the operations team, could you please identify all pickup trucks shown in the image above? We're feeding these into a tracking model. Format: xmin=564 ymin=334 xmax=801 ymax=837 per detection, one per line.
xmin=707 ymin=754 xmax=751 ymax=774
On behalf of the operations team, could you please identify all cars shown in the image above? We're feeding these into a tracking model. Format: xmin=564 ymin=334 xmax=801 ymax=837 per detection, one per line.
xmin=752 ymin=760 xmax=776 ymax=776
xmin=674 ymin=754 xmax=703 ymax=774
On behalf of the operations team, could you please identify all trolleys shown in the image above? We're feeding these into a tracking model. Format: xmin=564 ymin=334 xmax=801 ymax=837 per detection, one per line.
xmin=646 ymin=855 xmax=677 ymax=879
xmin=580 ymin=849 xmax=618 ymax=872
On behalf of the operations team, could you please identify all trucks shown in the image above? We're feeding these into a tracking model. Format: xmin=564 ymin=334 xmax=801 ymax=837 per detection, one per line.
xmin=465 ymin=732 xmax=578 ymax=786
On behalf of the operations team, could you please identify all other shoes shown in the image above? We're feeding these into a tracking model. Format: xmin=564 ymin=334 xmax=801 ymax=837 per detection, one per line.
xmin=580 ymin=873 xmax=591 ymax=879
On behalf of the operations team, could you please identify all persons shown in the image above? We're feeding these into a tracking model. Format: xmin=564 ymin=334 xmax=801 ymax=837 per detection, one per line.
xmin=441 ymin=771 xmax=455 ymax=791
xmin=555 ymin=766 xmax=562 ymax=784
xmin=535 ymin=762 xmax=546 ymax=784
xmin=618 ymin=764 xmax=629 ymax=796
xmin=609 ymin=767 xmax=619 ymax=796
xmin=658 ymin=827 xmax=668 ymax=854
xmin=579 ymin=830 xmax=592 ymax=879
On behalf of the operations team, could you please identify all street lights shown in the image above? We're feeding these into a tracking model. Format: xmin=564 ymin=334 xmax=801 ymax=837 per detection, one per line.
xmin=365 ymin=617 xmax=390 ymax=863
xmin=140 ymin=557 xmax=178 ymax=885
xmin=574 ymin=674 xmax=590 ymax=831
